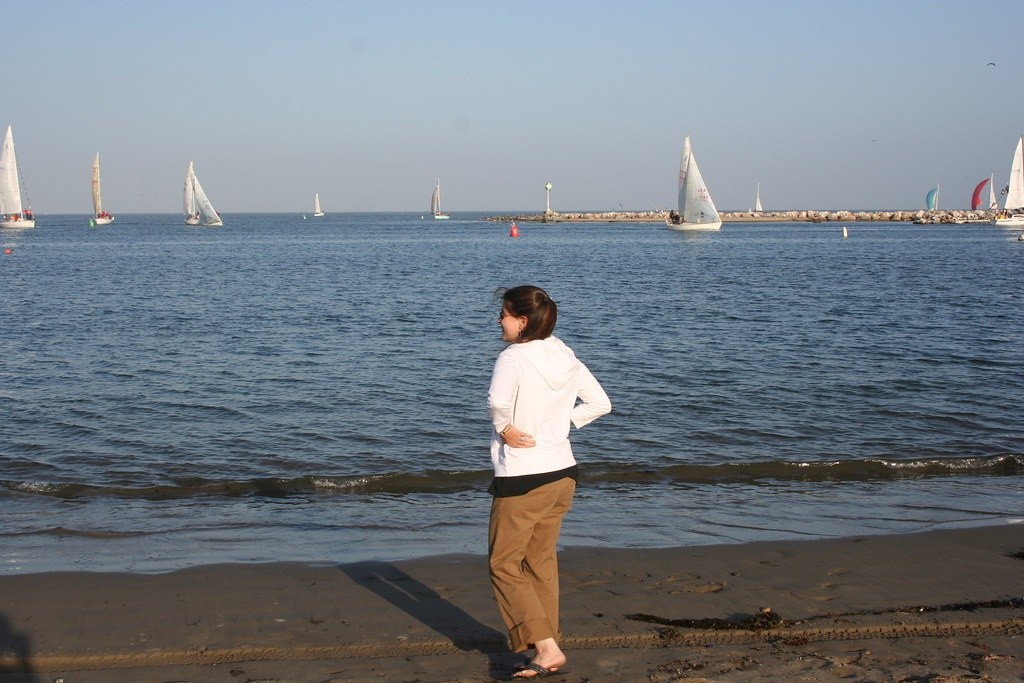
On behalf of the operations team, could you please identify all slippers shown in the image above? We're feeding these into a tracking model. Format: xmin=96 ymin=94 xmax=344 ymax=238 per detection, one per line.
xmin=512 ymin=656 xmax=573 ymax=681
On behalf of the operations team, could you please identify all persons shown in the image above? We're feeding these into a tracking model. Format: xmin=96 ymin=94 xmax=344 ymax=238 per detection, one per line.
xmin=98 ymin=210 xmax=111 ymax=220
xmin=486 ymin=286 xmax=612 ymax=680
xmin=3 ymin=208 xmax=32 ymax=222
xmin=670 ymin=209 xmax=681 ymax=225
xmin=186 ymin=211 xmax=200 ymax=220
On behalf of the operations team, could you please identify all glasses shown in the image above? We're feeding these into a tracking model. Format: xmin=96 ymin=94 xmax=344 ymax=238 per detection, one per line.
xmin=500 ymin=312 xmax=513 ymax=319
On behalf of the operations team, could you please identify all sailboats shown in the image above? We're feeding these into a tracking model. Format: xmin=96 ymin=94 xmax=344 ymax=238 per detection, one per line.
xmin=183 ymin=160 xmax=223 ymax=226
xmin=430 ymin=180 xmax=449 ymax=220
xmin=994 ymin=135 xmax=1024 ymax=231
xmin=91 ymin=151 xmax=115 ymax=225
xmin=313 ymin=193 xmax=324 ymax=217
xmin=664 ymin=136 xmax=723 ymax=231
xmin=0 ymin=124 xmax=35 ymax=229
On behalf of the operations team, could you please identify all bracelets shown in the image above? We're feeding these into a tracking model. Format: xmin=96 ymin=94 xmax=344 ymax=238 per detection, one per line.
xmin=499 ymin=425 xmax=513 ymax=437
